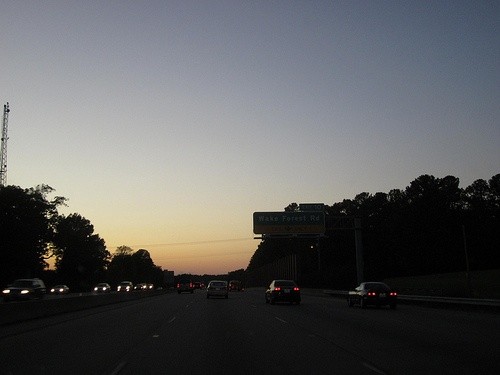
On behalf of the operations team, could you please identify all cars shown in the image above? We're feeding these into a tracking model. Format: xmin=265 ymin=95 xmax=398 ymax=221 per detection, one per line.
xmin=265 ymin=279 xmax=301 ymax=305
xmin=206 ymin=279 xmax=245 ymax=299
xmin=117 ymin=281 xmax=154 ymax=291
xmin=93 ymin=283 xmax=111 ymax=292
xmin=50 ymin=284 xmax=70 ymax=295
xmin=347 ymin=281 xmax=397 ymax=309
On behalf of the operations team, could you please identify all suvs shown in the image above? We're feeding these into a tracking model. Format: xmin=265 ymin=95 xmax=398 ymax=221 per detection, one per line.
xmin=176 ymin=278 xmax=205 ymax=292
xmin=2 ymin=278 xmax=46 ymax=301
